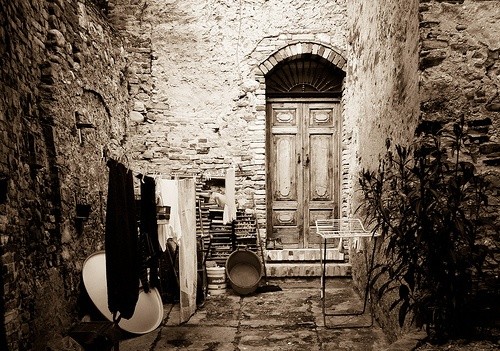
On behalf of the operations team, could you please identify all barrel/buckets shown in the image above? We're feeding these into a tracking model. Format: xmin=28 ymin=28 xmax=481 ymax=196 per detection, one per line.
xmin=206 ymin=265 xmax=226 ymax=297
xmin=226 ymin=248 xmax=263 ymax=296
